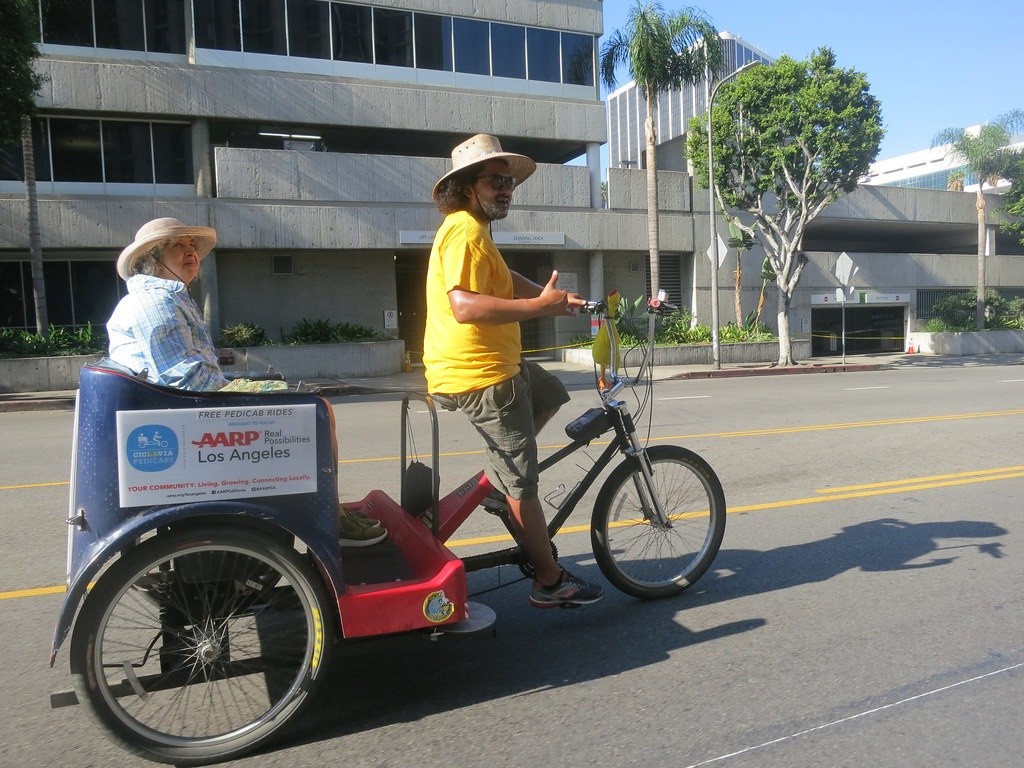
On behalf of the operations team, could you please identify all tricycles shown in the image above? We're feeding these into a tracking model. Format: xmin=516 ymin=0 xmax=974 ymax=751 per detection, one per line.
xmin=49 ymin=299 xmax=727 ymax=766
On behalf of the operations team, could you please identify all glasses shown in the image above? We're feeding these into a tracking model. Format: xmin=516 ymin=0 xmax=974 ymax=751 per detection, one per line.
xmin=478 ymin=175 xmax=518 ymax=192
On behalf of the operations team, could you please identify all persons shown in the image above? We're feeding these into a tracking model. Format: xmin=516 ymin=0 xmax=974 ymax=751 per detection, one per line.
xmin=421 ymin=134 xmax=604 ymax=607
xmin=105 ymin=215 xmax=390 ymax=546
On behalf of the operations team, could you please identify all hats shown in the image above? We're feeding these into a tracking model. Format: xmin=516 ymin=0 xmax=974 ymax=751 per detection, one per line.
xmin=116 ymin=217 xmax=216 ymax=281
xmin=433 ymin=134 xmax=536 ymax=201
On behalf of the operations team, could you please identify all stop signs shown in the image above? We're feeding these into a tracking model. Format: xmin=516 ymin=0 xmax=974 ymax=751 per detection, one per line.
xmin=824 ymin=296 xmax=828 ymax=302
xmin=896 ymin=296 xmax=900 ymax=300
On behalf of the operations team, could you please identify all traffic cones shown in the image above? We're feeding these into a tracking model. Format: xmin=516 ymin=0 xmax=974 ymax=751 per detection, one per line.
xmin=906 ymin=337 xmax=915 ymax=353
xmin=403 ymin=351 xmax=414 ymax=372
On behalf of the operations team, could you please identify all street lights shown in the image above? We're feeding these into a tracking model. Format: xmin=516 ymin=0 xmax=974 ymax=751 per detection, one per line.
xmin=708 ymin=60 xmax=762 ymax=369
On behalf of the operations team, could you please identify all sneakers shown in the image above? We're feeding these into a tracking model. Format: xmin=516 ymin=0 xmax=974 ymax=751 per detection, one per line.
xmin=530 ymin=570 xmax=605 ymax=609
xmin=345 ymin=510 xmax=380 ymax=528
xmin=479 ymin=489 xmax=509 ymax=511
xmin=339 ymin=516 xmax=387 ymax=546
